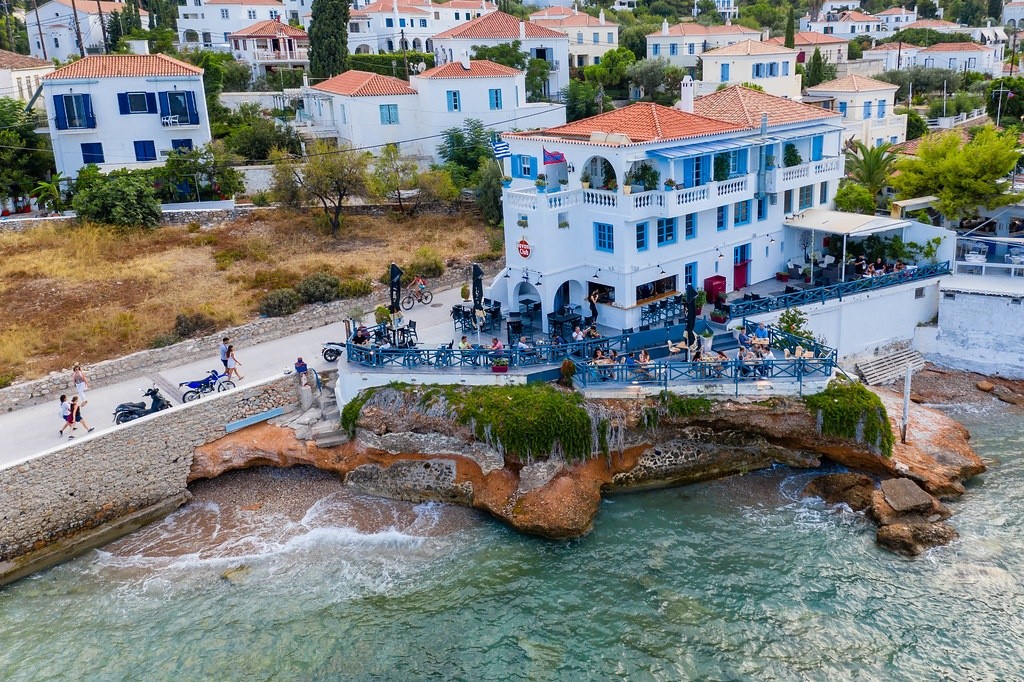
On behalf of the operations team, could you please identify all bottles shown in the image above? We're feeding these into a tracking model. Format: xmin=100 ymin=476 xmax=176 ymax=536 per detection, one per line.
xmin=542 ymin=338 xmax=544 ymax=344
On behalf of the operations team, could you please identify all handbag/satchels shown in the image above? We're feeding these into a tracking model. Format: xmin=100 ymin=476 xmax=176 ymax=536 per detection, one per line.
xmin=67 ymin=413 xmax=74 ymax=424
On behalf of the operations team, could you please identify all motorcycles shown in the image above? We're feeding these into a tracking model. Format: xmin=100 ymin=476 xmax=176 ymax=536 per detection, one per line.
xmin=322 ymin=340 xmax=347 ymax=362
xmin=113 ymin=383 xmax=174 ymax=426
xmin=178 ymin=369 xmax=235 ymax=402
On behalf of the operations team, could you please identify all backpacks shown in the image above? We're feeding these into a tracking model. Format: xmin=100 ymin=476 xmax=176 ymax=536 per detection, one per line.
xmin=418 ymin=278 xmax=425 ymax=285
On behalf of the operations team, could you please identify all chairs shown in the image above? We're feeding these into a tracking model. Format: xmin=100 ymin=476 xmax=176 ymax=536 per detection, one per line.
xmin=509 ymin=302 xmax=635 ymax=380
xmin=814 ymin=276 xmax=832 ymax=298
xmin=965 ymin=251 xmax=1024 ymax=278
xmin=639 ymin=333 xmax=822 ymax=380
xmin=358 ymin=295 xmax=507 ymax=366
xmin=639 ymin=286 xmax=805 ymax=332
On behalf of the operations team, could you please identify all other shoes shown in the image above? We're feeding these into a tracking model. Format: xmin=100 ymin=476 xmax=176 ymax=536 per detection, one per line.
xmin=73 ymin=428 xmax=77 ymax=430
xmin=69 ymin=436 xmax=75 ymax=440
xmin=60 ymin=431 xmax=62 ymax=434
xmin=88 ymin=428 xmax=94 ymax=432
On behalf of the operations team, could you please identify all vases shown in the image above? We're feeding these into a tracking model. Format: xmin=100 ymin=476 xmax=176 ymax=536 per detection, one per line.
xmin=492 ymin=363 xmax=509 ymax=372
xmin=561 ymin=376 xmax=570 ymax=387
xmin=767 ymin=163 xmax=776 ymax=170
xmin=501 ymin=180 xmax=673 ymax=194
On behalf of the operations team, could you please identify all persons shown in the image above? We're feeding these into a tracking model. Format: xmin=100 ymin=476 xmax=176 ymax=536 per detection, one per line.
xmin=355 ymin=307 xmax=404 ymax=362
xmin=691 ymin=322 xmax=776 ymax=379
xmin=407 ymin=275 xmax=426 ymax=300
xmin=220 ymin=337 xmax=244 ymax=385
xmin=588 ymin=290 xmax=599 ymax=322
xmin=457 ymin=323 xmax=599 ymax=366
xmin=638 ymin=283 xmax=654 ymax=298
xmin=854 ymin=253 xmax=913 ymax=285
xmin=72 ymin=366 xmax=89 ymax=407
xmin=57 ymin=394 xmax=94 ymax=440
xmin=592 ymin=348 xmax=650 ymax=380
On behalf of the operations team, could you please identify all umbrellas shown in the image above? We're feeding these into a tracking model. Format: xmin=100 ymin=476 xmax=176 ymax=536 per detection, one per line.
xmin=473 ymin=263 xmax=486 ymax=347
xmin=388 ymin=263 xmax=403 ymax=346
xmin=685 ymin=283 xmax=699 ymax=362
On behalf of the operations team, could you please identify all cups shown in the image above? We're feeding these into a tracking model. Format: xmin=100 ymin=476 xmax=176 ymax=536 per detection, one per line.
xmin=546 ymin=342 xmax=551 ymax=344
xmin=703 ymin=357 xmax=713 ymax=361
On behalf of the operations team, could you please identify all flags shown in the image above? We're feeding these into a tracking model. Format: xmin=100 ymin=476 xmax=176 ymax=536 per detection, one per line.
xmin=491 ymin=142 xmax=512 ymax=159
xmin=543 ymin=149 xmax=565 ymax=166
xmin=1008 ymin=92 xmax=1014 ymax=97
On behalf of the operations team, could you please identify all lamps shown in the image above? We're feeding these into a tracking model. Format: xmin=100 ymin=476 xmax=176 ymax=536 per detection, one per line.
xmin=503 ymin=233 xmax=776 ymax=286
xmin=568 ymin=162 xmax=575 ymax=173
xmin=770 ymin=193 xmax=778 ymax=205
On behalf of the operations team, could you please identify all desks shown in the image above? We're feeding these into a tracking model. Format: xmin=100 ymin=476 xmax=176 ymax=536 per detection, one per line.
xmin=364 ymin=298 xmax=615 ymax=378
xmin=642 ymin=365 xmax=655 ymax=383
xmin=636 ymin=360 xmax=657 ymax=376
xmin=704 ymin=283 xmax=812 ymax=377
xmin=594 ymin=358 xmax=616 ymax=382
xmin=675 ymin=341 xmax=689 ymax=362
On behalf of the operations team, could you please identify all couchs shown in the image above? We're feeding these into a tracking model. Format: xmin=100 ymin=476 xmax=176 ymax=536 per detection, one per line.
xmin=787 ymin=251 xmax=835 ymax=280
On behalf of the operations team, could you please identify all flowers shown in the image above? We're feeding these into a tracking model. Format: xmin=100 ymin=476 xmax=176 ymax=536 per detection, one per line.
xmin=492 ymin=359 xmax=505 ymax=366
xmin=766 ymin=154 xmax=777 ymax=165
xmin=561 ymin=359 xmax=576 ymax=377
xmin=501 ymin=158 xmax=675 ymax=187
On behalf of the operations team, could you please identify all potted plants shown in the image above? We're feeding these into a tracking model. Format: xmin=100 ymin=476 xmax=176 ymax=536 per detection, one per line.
xmin=374 ymin=305 xmax=392 ymax=325
xmin=776 ymin=255 xmax=817 ymax=282
xmin=461 ymin=283 xmax=470 ymax=300
xmin=694 ymin=290 xmax=745 ymax=352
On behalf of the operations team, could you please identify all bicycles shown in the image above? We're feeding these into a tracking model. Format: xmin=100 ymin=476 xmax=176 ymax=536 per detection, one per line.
xmin=402 ymin=288 xmax=433 ymax=310
